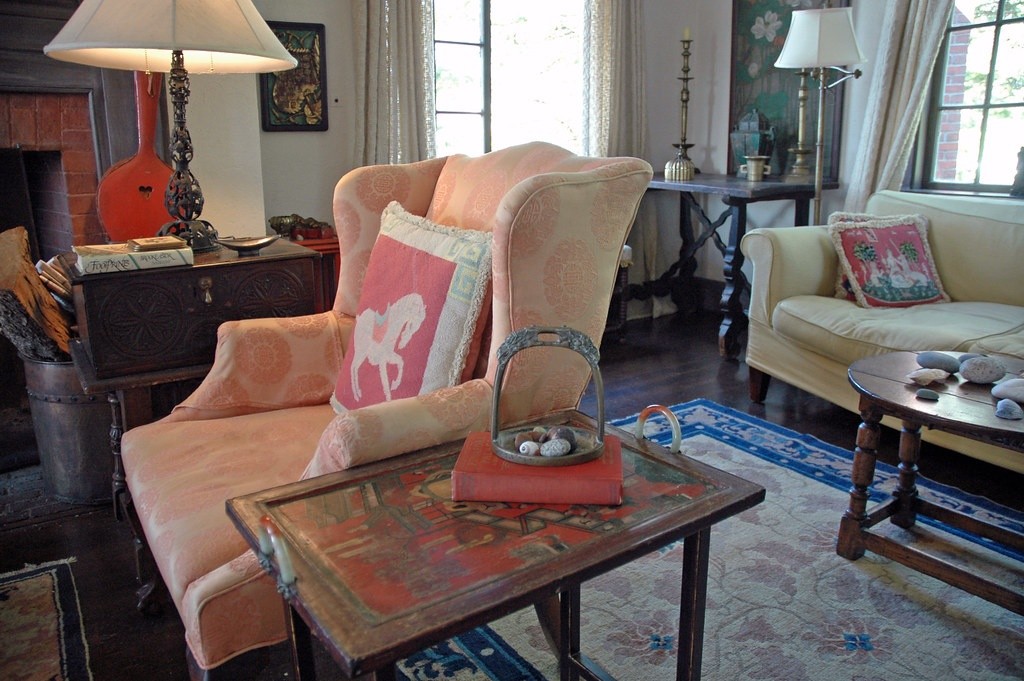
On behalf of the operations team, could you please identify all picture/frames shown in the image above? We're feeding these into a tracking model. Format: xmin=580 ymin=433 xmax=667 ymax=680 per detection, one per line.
xmin=727 ymin=0 xmax=851 ymax=179
xmin=260 ymin=20 xmax=329 ymax=132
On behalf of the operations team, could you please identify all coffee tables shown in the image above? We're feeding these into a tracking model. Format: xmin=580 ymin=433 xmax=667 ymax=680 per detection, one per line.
xmin=837 ymin=351 xmax=1024 ymax=618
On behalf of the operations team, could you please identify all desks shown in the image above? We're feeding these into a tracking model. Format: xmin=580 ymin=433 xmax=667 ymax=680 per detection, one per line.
xmin=285 ymin=233 xmax=338 ymax=295
xmin=643 ymin=171 xmax=840 ymax=362
xmin=224 ymin=408 xmax=766 ymax=681
xmin=56 ymin=238 xmax=323 ymax=625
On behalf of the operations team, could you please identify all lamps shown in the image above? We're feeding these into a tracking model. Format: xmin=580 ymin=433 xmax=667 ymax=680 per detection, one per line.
xmin=773 ymin=7 xmax=871 ymax=184
xmin=42 ymin=0 xmax=300 ymax=253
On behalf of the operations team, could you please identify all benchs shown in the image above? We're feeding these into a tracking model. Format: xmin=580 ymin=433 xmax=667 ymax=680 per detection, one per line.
xmin=740 ymin=188 xmax=1024 ymax=474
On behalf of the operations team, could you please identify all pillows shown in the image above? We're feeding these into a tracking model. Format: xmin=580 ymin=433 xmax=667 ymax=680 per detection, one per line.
xmin=829 ymin=210 xmax=951 ymax=308
xmin=328 ymin=201 xmax=493 ymax=416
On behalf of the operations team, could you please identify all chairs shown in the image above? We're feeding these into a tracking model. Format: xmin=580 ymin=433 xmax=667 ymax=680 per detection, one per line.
xmin=114 ymin=148 xmax=653 ymax=681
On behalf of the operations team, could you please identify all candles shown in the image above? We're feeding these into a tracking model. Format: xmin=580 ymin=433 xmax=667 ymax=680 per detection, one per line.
xmin=683 ymin=25 xmax=690 ymax=40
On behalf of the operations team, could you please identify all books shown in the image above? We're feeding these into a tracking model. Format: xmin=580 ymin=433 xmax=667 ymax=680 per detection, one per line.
xmin=452 ymin=430 xmax=623 ymax=505
xmin=72 ymin=243 xmax=194 ymax=274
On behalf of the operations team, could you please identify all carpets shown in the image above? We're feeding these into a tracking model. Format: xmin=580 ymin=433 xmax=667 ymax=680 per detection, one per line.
xmin=0 ymin=556 xmax=95 ymax=681
xmin=393 ymin=397 xmax=1024 ymax=681
